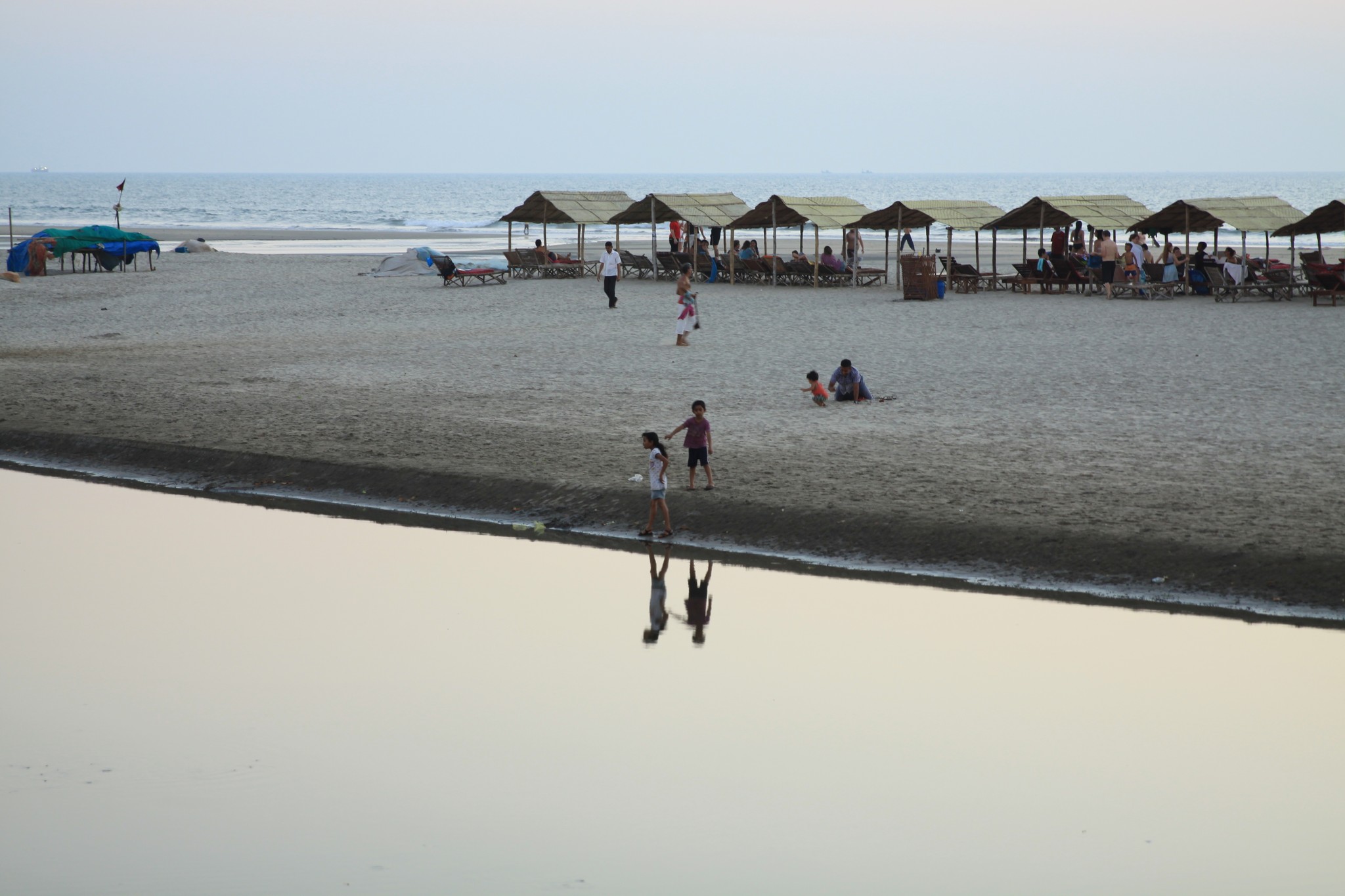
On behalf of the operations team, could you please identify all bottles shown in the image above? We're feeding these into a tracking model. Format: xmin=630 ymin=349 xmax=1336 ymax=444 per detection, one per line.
xmin=1150 ymin=577 xmax=1165 ymax=583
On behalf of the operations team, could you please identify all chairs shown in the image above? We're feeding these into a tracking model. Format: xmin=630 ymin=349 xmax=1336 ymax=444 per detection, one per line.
xmin=505 ymin=246 xmax=1345 ymax=307
xmin=428 ymin=255 xmax=508 ymax=288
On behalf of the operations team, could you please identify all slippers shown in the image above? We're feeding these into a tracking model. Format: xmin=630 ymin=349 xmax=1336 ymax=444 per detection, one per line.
xmin=881 ymin=395 xmax=893 ymax=400
xmin=891 ymin=394 xmax=897 ymax=399
xmin=684 ymin=488 xmax=696 ymax=491
xmin=704 ymin=485 xmax=713 ymax=490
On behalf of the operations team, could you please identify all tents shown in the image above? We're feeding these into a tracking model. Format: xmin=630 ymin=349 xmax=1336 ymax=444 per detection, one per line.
xmin=841 ymin=200 xmax=1008 ymax=291
xmin=980 ymin=195 xmax=1158 ymax=294
xmin=606 ymin=191 xmax=753 ymax=282
xmin=1270 ymin=200 xmax=1345 ymax=266
xmin=1126 ymin=194 xmax=1307 ymax=301
xmin=497 ymin=190 xmax=636 ymax=278
xmin=724 ymin=196 xmax=876 ymax=287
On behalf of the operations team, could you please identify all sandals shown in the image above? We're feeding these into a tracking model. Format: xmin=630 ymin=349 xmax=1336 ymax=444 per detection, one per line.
xmin=658 ymin=529 xmax=673 ymax=538
xmin=639 ymin=528 xmax=653 ymax=536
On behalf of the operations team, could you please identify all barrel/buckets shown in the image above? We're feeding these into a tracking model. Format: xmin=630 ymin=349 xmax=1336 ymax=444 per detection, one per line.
xmin=175 ymin=246 xmax=188 ymax=253
xmin=937 ymin=280 xmax=945 ymax=299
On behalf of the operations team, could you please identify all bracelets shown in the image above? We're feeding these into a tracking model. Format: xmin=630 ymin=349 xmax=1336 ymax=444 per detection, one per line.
xmin=854 ymin=400 xmax=858 ymax=403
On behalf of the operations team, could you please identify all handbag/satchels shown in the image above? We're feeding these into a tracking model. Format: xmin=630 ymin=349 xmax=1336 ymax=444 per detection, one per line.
xmin=440 ymin=255 xmax=456 ymax=280
xmin=426 ymin=256 xmax=433 ymax=267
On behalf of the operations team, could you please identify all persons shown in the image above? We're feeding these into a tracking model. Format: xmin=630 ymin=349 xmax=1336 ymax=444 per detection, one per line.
xmin=524 ymin=222 xmax=529 ymax=234
xmin=792 ymin=229 xmax=915 ymax=273
xmin=533 ymin=239 xmax=571 ymax=261
xmin=637 ymin=539 xmax=713 ymax=644
xmin=597 ymin=241 xmax=622 ymax=309
xmin=800 ymin=371 xmax=828 ymax=406
xmin=829 ymin=359 xmax=875 ymax=405
xmin=675 ymin=263 xmax=696 ymax=347
xmin=1038 ymin=221 xmax=1237 ymax=300
xmin=638 ymin=400 xmax=715 ymax=538
xmin=669 ymin=220 xmax=760 ymax=281
